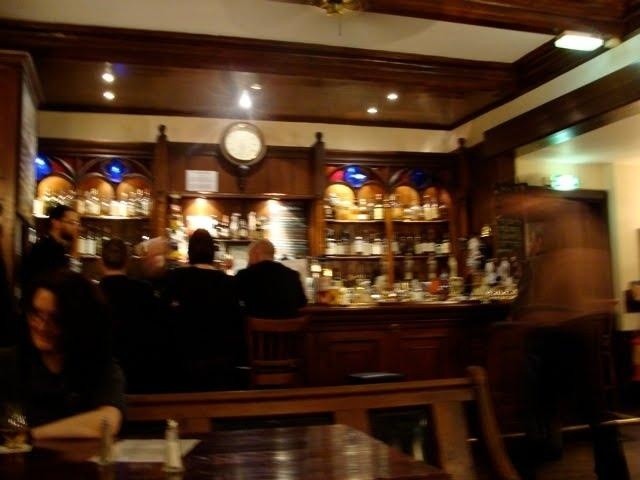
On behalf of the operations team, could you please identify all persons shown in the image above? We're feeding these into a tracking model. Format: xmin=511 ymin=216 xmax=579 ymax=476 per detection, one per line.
xmin=134 ymin=238 xmax=181 ymax=299
xmin=15 ymin=203 xmax=88 ymax=420
xmin=150 ymin=228 xmax=249 ymax=390
xmin=230 ymin=238 xmax=311 ymax=390
xmin=83 ymin=235 xmax=156 ymax=396
xmin=1 ymin=266 xmax=131 ymax=444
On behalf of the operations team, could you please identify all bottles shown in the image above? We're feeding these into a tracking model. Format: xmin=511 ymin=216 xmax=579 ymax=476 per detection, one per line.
xmin=27 ymin=222 xmax=112 ymax=258
xmin=219 ymin=211 xmax=257 ymax=240
xmin=322 ymin=192 xmax=452 ymax=256
xmin=44 ymin=186 xmax=154 ymax=217
xmin=95 ymin=417 xmax=117 ymax=479
xmin=161 ymin=421 xmax=187 ymax=479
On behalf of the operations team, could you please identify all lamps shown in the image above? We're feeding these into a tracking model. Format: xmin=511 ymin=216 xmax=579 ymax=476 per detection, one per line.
xmin=311 ymin=0 xmax=363 ymax=15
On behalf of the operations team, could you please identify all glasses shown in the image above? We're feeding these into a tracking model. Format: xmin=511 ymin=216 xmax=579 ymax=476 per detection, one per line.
xmin=26 ymin=311 xmax=58 ymax=328
xmin=60 ymin=219 xmax=80 ymax=225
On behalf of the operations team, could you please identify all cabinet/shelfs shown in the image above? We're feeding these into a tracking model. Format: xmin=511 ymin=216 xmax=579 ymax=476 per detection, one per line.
xmin=315 ymin=147 xmax=463 ymax=288
xmin=159 ymin=127 xmax=320 ymax=277
xmin=34 ymin=137 xmax=158 ymax=274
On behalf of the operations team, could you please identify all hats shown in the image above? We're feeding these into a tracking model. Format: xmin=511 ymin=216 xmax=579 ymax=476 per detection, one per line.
xmin=189 ymin=228 xmax=215 ymax=263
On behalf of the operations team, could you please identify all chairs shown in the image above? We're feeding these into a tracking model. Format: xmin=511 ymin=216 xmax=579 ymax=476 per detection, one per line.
xmin=245 ymin=316 xmax=308 ymax=388
